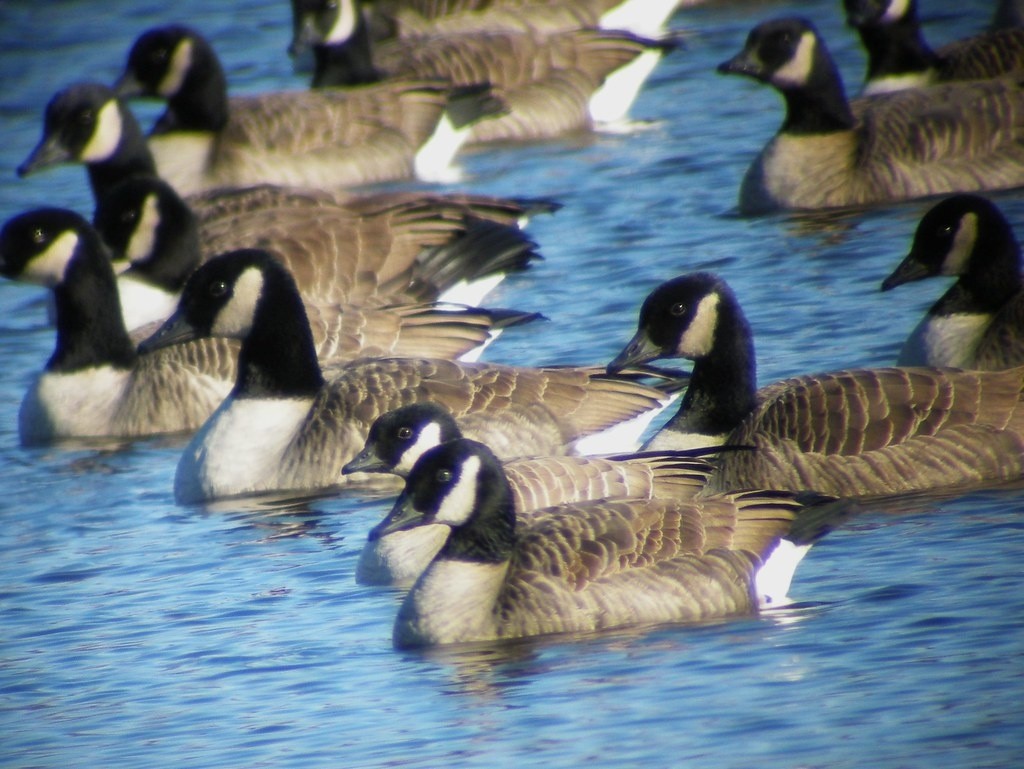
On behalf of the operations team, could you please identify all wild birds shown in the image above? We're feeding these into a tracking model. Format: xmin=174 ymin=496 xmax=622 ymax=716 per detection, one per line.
xmin=0 ymin=1 xmax=1024 ymax=650
